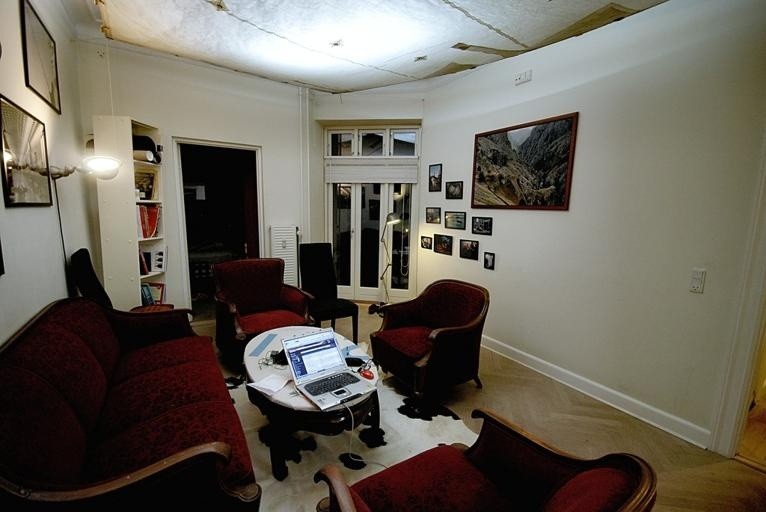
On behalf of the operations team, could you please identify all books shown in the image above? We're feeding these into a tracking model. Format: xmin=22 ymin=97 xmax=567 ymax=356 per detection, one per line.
xmin=134 ymin=167 xmax=168 ymax=306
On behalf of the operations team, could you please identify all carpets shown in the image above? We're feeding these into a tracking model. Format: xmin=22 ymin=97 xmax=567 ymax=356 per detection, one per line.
xmin=221 ymin=352 xmax=478 ymax=512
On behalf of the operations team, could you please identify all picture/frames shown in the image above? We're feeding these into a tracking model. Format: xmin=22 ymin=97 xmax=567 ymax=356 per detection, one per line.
xmin=19 ymin=0 xmax=62 ymax=114
xmin=0 ymin=93 xmax=53 ymax=208
xmin=420 ymin=162 xmax=496 ymax=270
xmin=469 ymin=108 xmax=579 ymax=210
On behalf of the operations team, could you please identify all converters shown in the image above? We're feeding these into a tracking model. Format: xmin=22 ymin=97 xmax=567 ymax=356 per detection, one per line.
xmin=346 ymin=358 xmax=365 ymax=366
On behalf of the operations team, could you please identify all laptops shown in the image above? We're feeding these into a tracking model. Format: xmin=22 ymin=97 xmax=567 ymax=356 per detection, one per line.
xmin=281 ymin=327 xmax=379 ymax=411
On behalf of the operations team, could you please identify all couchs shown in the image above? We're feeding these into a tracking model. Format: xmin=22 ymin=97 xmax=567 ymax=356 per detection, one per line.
xmin=0 ymin=297 xmax=262 ymax=512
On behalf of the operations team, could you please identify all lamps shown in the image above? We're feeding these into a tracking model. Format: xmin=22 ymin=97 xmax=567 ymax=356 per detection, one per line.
xmin=49 ymin=152 xmax=120 ymax=181
xmin=380 ymin=212 xmax=400 ymax=305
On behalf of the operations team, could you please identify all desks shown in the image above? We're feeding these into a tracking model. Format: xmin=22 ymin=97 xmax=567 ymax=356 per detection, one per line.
xmin=128 ymin=303 xmax=172 ymax=313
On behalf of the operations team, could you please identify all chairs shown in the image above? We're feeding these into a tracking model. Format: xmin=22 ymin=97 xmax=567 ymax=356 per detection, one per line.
xmin=208 ymin=254 xmax=316 ymax=368
xmin=69 ymin=248 xmax=126 ymax=315
xmin=370 ymin=279 xmax=490 ymax=402
xmin=313 ymin=406 xmax=657 ymax=511
xmin=298 ymin=242 xmax=358 ymax=344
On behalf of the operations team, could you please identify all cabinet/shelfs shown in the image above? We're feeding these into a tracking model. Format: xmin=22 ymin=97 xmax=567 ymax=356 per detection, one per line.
xmin=92 ymin=114 xmax=166 ymax=313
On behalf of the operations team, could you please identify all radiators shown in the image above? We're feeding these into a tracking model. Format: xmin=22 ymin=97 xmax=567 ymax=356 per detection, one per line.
xmin=269 ymin=225 xmax=300 ymax=289
